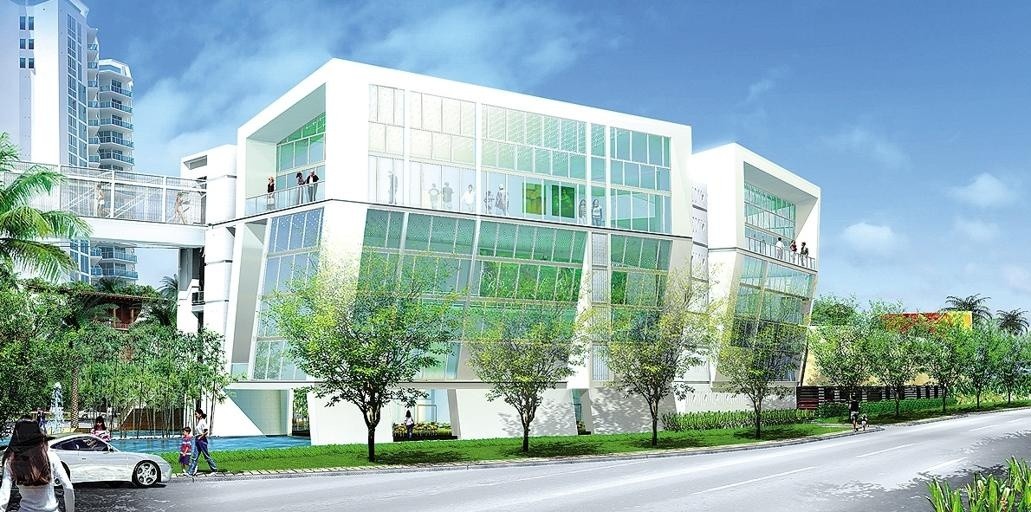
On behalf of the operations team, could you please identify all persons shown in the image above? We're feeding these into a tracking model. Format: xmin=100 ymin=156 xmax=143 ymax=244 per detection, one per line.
xmin=848 ymin=395 xmax=859 ymax=434
xmin=174 ymin=191 xmax=186 ymax=224
xmin=81 ymin=403 xmax=127 ymax=428
xmin=296 ymin=172 xmax=304 ymax=204
xmin=429 ymin=183 xmax=440 ymax=210
xmin=38 ymin=409 xmax=47 ymax=435
xmin=592 ymin=199 xmax=603 ymax=226
xmin=95 ymin=184 xmax=106 ymax=218
xmin=789 ymin=240 xmax=797 ymax=263
xmin=183 ymin=408 xmax=219 ymax=477
xmin=557 ymin=189 xmax=571 ymax=215
xmin=0 ymin=415 xmax=76 ymax=512
xmin=579 ymin=199 xmax=586 ymax=224
xmin=861 ymin=413 xmax=868 ymax=430
xmin=460 ymin=184 xmax=474 ymax=213
xmin=36 ymin=406 xmax=41 ymax=425
xmin=484 ymin=190 xmax=495 ymax=214
xmin=441 ymin=182 xmax=455 ymax=209
xmin=267 ymin=176 xmax=277 ymax=210
xmin=387 ymin=172 xmax=398 ymax=205
xmin=496 ymin=183 xmax=510 ymax=216
xmin=800 ymin=241 xmax=808 ymax=267
xmin=178 ymin=427 xmax=192 ymax=477
xmin=775 ymin=236 xmax=784 ymax=260
xmin=405 ymin=410 xmax=414 ymax=439
xmin=306 ymin=170 xmax=319 ymax=201
xmin=90 ymin=416 xmax=111 ymax=451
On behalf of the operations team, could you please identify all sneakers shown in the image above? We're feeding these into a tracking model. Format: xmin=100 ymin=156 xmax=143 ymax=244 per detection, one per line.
xmin=179 ymin=465 xmax=199 ymax=477
xmin=208 ymin=471 xmax=219 ymax=476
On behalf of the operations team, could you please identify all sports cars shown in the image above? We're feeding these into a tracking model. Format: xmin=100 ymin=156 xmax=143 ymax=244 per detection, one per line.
xmin=0 ymin=434 xmax=173 ymax=491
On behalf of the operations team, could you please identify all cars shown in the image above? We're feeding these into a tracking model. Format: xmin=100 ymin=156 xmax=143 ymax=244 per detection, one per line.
xmin=0 ymin=438 xmax=12 ymax=479
